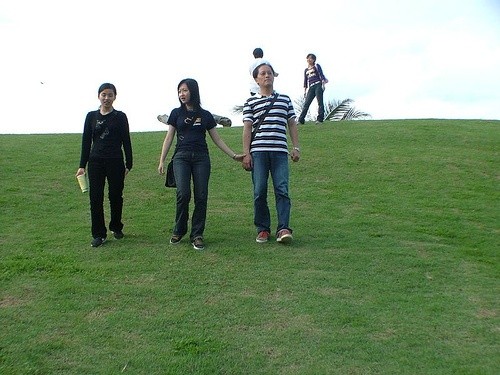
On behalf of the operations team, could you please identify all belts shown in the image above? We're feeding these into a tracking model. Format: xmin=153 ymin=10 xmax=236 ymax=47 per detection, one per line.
xmin=311 ymin=81 xmax=320 ymax=87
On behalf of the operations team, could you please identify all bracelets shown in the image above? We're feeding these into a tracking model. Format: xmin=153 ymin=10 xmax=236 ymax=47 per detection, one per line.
xmin=292 ymin=147 xmax=299 ymax=151
xmin=233 ymin=154 xmax=238 ymax=159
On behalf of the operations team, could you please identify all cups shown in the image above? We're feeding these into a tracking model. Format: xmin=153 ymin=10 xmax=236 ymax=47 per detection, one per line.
xmin=77 ymin=175 xmax=88 ymax=193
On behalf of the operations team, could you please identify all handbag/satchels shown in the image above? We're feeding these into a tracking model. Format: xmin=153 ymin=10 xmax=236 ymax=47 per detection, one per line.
xmin=165 ymin=162 xmax=177 ymax=187
xmin=315 ymin=64 xmax=328 ymax=83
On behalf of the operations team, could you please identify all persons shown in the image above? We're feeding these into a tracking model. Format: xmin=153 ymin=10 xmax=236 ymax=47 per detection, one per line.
xmin=297 ymin=53 xmax=327 ymax=124
xmin=242 ymin=62 xmax=301 ymax=244
xmin=76 ymin=83 xmax=133 ymax=248
xmin=249 ymin=48 xmax=279 ymax=97
xmin=158 ymin=77 xmax=246 ymax=250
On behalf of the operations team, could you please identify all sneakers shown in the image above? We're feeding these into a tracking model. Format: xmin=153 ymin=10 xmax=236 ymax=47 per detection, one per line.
xmin=191 ymin=237 xmax=204 ymax=250
xmin=276 ymin=229 xmax=293 ymax=242
xmin=111 ymin=230 xmax=124 ymax=240
xmin=170 ymin=232 xmax=185 ymax=245
xmin=255 ymin=231 xmax=269 ymax=243
xmin=90 ymin=235 xmax=106 ymax=247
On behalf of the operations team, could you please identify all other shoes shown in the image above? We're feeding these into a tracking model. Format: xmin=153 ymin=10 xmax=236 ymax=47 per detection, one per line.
xmin=314 ymin=120 xmax=323 ymax=125
xmin=296 ymin=122 xmax=302 ymax=125
